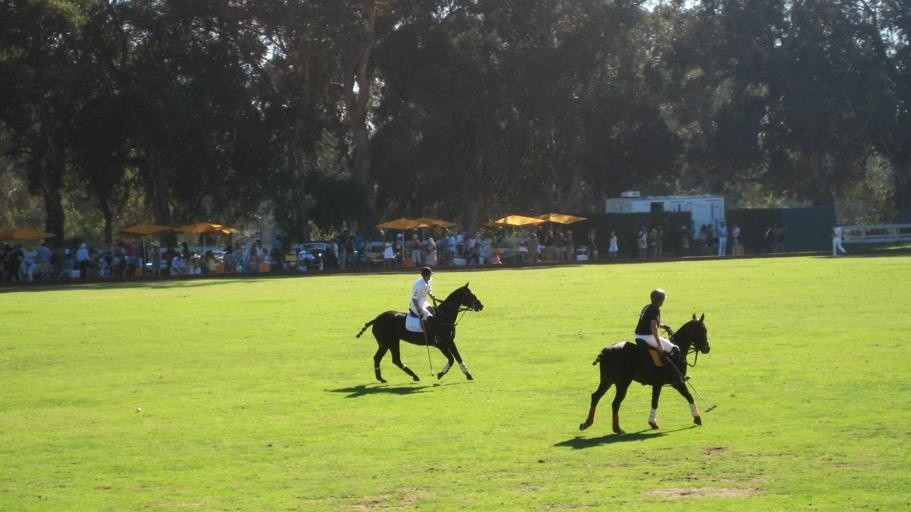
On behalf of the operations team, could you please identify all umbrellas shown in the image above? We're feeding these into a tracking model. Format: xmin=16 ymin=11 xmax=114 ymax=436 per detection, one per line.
xmin=536 ymin=212 xmax=588 ymax=237
xmin=482 ymin=213 xmax=545 ymax=233
xmin=375 ymin=217 xmax=430 ymax=260
xmin=410 ymin=216 xmax=458 ymax=248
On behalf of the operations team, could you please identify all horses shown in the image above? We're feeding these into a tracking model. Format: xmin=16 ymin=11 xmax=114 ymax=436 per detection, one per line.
xmin=357 ymin=282 xmax=483 ymax=383
xmin=580 ymin=312 xmax=710 ymax=433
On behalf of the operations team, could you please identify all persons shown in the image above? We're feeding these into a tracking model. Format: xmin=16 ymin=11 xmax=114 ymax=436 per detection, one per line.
xmin=407 ymin=266 xmax=435 ymax=345
xmin=1 ymin=238 xmax=107 ymax=285
xmin=111 ymin=237 xmax=194 ymax=280
xmin=633 ymin=288 xmax=692 ymax=384
xmin=831 ymin=223 xmax=849 ymax=258
xmin=608 ymin=229 xmax=619 ymax=262
xmin=382 ymin=223 xmax=599 ymax=270
xmin=246 ymin=229 xmax=356 ymax=274
xmin=636 ymin=223 xmax=743 ymax=258
xmin=765 ymin=225 xmax=777 ymax=256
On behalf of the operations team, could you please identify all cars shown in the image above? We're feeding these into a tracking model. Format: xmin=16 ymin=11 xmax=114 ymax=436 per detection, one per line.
xmin=363 ymin=241 xmax=386 ymax=266
xmin=294 ymin=241 xmax=335 ymax=271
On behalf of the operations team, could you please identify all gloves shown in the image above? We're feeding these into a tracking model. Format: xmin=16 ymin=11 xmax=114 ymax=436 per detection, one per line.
xmin=663 ymin=325 xmax=672 ymax=335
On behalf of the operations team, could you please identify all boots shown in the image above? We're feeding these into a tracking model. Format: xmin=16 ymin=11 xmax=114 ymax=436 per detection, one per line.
xmin=673 ymin=347 xmax=689 ymax=385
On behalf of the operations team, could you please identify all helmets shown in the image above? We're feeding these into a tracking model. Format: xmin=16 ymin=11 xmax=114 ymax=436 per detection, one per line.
xmin=421 ymin=267 xmax=434 ymax=274
xmin=651 ymin=290 xmax=665 ymax=303
